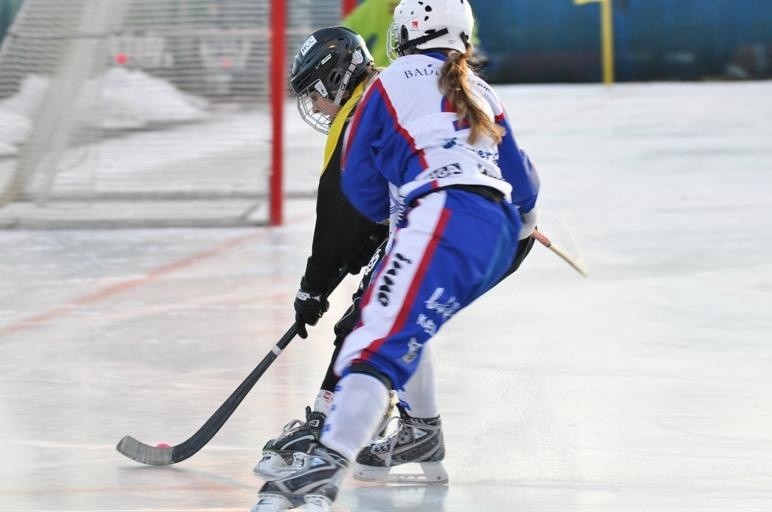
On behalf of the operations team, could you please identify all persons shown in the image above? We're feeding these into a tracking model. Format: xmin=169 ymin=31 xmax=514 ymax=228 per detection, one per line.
xmin=253 ymin=25 xmax=446 ymax=476
xmin=258 ymin=0 xmax=541 ymax=509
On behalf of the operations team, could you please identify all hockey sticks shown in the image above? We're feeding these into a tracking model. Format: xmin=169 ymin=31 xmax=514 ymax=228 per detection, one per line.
xmin=117 ymin=267 xmax=349 ymax=468
xmin=532 ymin=227 xmax=584 ymax=276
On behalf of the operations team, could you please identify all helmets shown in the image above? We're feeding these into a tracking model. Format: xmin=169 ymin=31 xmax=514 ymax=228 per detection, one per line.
xmin=386 ymin=0 xmax=474 ymax=63
xmin=289 ymin=26 xmax=375 ymax=135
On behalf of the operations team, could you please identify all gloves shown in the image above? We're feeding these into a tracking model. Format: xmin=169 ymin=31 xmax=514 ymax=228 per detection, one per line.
xmin=294 ymin=278 xmax=330 ymax=338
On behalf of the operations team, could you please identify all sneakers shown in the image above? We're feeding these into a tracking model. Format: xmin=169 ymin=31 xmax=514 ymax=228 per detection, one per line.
xmin=259 ymin=405 xmax=444 ymax=506
